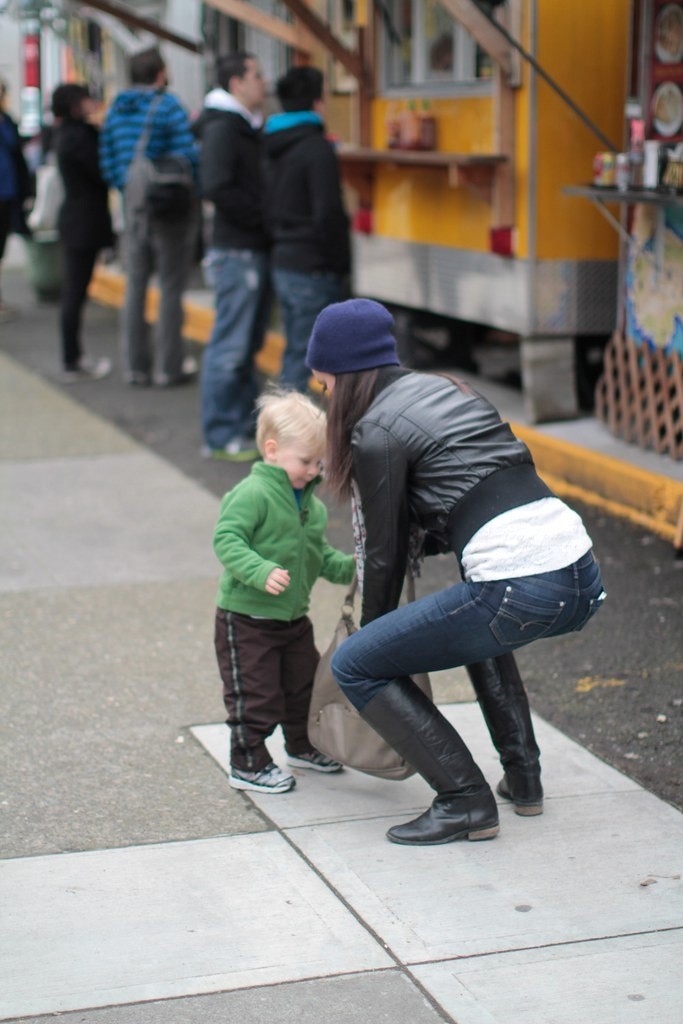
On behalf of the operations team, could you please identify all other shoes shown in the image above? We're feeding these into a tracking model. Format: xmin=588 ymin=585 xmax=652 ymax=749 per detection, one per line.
xmin=129 ymin=371 xmax=151 ymax=384
xmin=155 ymin=355 xmax=200 ymax=385
xmin=60 ymin=358 xmax=106 ymax=376
xmin=64 ymin=363 xmax=111 ymax=382
xmin=203 ymin=436 xmax=259 ymax=463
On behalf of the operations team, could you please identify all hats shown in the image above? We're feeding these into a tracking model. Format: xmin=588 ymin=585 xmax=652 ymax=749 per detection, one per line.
xmin=305 ymin=298 xmax=400 ymax=374
xmin=52 ymin=82 xmax=89 ymax=116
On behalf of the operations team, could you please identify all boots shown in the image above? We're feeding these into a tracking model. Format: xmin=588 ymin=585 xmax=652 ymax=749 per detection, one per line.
xmin=466 ymin=653 xmax=544 ymax=816
xmin=360 ymin=675 xmax=500 ymax=846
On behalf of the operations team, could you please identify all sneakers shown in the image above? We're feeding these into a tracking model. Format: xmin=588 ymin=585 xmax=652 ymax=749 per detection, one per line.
xmin=229 ymin=763 xmax=296 ymax=794
xmin=288 ymin=748 xmax=343 ymax=772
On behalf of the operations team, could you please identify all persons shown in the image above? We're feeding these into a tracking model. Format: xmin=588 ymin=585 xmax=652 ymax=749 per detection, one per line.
xmin=0 ymin=79 xmax=30 ymax=266
xmin=53 ymin=82 xmax=119 ymax=384
xmin=187 ymin=52 xmax=274 ymax=464
xmin=259 ymin=64 xmax=355 ymax=394
xmin=37 ymin=80 xmax=70 ymax=298
xmin=213 ymin=377 xmax=357 ymax=794
xmin=98 ymin=47 xmax=200 ymax=388
xmin=305 ymin=296 xmax=609 ymax=846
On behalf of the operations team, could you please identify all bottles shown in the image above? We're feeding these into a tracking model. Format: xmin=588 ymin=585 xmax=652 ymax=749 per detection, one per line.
xmin=383 ymin=97 xmax=437 ymax=151
xmin=594 ymin=137 xmax=659 ymax=187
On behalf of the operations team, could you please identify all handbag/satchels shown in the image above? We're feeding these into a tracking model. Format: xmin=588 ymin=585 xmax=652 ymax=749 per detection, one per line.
xmin=28 ymin=150 xmax=66 ymax=242
xmin=308 ymin=620 xmax=434 ymax=781
xmin=124 ymin=157 xmax=195 ymax=228
